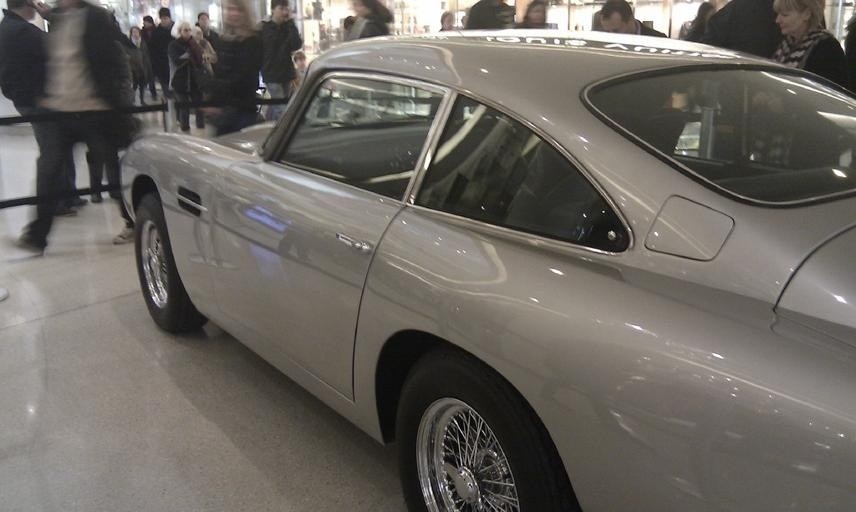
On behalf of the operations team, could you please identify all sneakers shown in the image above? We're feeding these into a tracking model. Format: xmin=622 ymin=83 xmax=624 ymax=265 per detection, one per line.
xmin=112 ymin=227 xmax=134 ymax=245
xmin=52 ymin=189 xmax=123 ymax=217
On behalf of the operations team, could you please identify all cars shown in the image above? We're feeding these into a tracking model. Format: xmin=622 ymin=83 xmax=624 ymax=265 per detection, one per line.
xmin=117 ymin=28 xmax=856 ymax=512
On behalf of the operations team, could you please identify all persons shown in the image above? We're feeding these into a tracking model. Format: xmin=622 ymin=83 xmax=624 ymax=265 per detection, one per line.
xmin=0 ymin=0 xmax=144 ymax=262
xmin=0 ymin=0 xmax=89 ymax=219
xmin=432 ymin=0 xmax=856 ymax=171
xmin=126 ymin=1 xmax=394 ymax=136
xmin=86 ymin=139 xmax=121 ymax=205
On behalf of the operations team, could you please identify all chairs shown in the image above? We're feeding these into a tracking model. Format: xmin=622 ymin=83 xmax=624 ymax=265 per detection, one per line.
xmin=504 ymin=140 xmax=604 ymax=235
xmin=423 ymin=119 xmax=531 ymax=210
xmin=636 ymin=106 xmax=686 ymax=155
